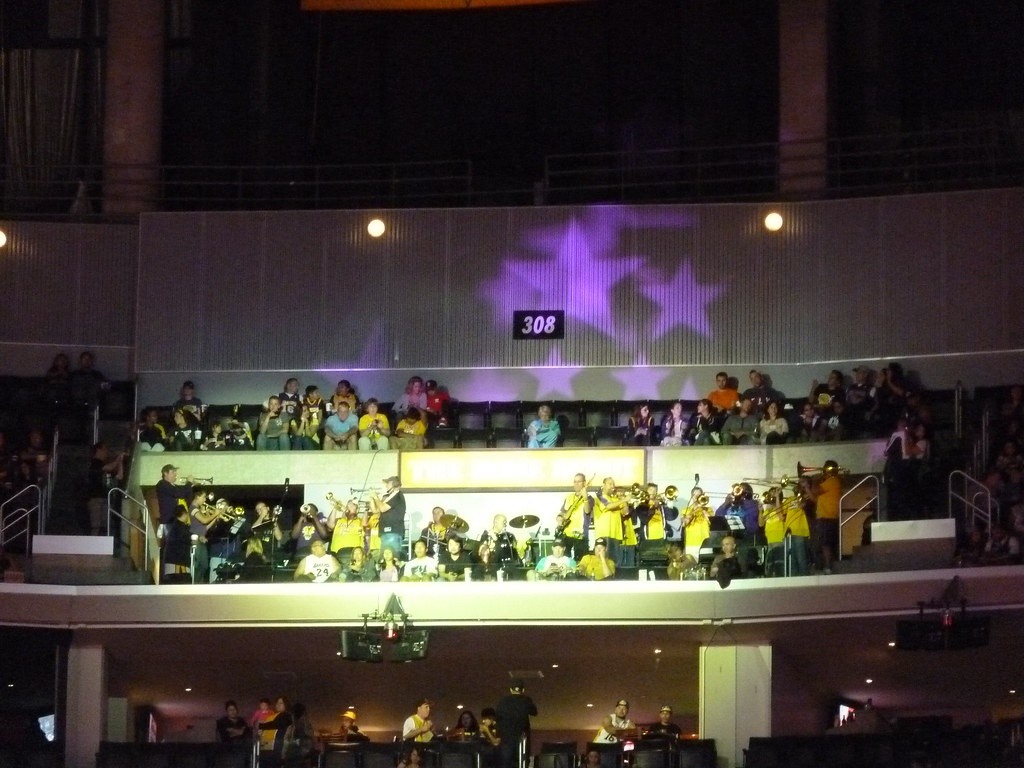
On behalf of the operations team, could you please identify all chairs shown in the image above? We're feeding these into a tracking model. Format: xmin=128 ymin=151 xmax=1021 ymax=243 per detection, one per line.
xmin=143 ymin=398 xmax=702 ymax=448
xmin=160 ymin=515 xmax=818 ymax=586
xmin=586 ymin=741 xmax=624 ymax=768
xmin=539 ymin=740 xmax=578 ymax=763
xmin=318 ymin=728 xmax=348 ymax=753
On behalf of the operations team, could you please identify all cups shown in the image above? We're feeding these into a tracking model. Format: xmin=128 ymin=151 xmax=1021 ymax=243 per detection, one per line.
xmin=464 ymin=567 xmax=472 ymax=581
xmin=438 ymin=564 xmax=445 ymax=577
xmin=496 ymin=570 xmax=505 ymax=582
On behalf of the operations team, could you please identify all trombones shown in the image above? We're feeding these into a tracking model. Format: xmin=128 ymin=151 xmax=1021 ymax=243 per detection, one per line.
xmin=742 ymin=474 xmax=800 ymax=491
xmin=763 ymin=493 xmax=810 ymax=521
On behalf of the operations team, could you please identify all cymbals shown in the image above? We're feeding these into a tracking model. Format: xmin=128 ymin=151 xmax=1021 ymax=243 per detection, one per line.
xmin=439 ymin=514 xmax=470 ymax=534
xmin=509 ymin=514 xmax=540 ymax=529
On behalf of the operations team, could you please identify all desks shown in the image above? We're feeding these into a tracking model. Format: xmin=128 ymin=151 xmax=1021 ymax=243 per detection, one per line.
xmin=323 ymin=742 xmax=491 ymax=768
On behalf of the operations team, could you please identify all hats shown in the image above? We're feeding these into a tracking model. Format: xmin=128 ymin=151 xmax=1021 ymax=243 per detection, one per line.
xmin=161 ymin=464 xmax=180 ymax=472
xmin=595 ymin=537 xmax=607 ymax=547
xmin=511 ymin=682 xmax=523 ymax=692
xmin=615 ymin=699 xmax=629 ymax=709
xmin=382 ymin=476 xmax=401 ymax=488
xmin=552 ymin=538 xmax=565 ymax=547
xmin=183 ymin=381 xmax=194 ymax=389
xmin=340 ymin=710 xmax=356 ymax=720
xmin=425 ymin=380 xmax=437 ymax=391
xmin=852 ymin=365 xmax=871 ymax=374
xmin=660 ymin=705 xmax=672 ymax=712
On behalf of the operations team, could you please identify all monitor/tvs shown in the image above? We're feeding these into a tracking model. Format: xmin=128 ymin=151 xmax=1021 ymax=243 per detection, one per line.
xmin=389 ymin=632 xmax=429 ymax=663
xmin=342 ymin=630 xmax=383 ymax=663
xmin=895 ymin=614 xmax=990 ymax=652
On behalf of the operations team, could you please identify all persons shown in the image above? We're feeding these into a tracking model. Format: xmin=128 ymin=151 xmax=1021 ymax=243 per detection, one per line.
xmin=593 ymin=699 xmax=637 ymax=743
xmin=648 ymin=705 xmax=683 ymax=733
xmin=402 ymin=698 xmax=436 ymax=742
xmin=154 ymin=459 xmax=843 ymax=584
xmin=479 ymin=680 xmax=538 ymax=768
xmin=0 ymin=351 xmax=130 ymax=535
xmin=622 ymin=362 xmax=936 ymax=545
xmin=962 ymin=383 xmax=1024 ymax=557
xmin=580 ymin=748 xmax=603 ymax=768
xmin=527 ymin=404 xmax=561 ymax=447
xmin=398 ymin=746 xmax=425 ymax=768
xmin=216 ymin=697 xmax=324 ymax=768
xmin=126 ymin=375 xmax=452 ymax=452
xmin=338 ymin=710 xmax=367 ymax=735
xmin=456 ymin=710 xmax=479 ymax=736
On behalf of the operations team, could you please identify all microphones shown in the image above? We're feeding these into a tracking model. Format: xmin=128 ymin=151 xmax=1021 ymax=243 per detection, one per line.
xmin=695 ymin=474 xmax=699 ymax=485
xmin=503 ymin=521 xmax=506 ymax=531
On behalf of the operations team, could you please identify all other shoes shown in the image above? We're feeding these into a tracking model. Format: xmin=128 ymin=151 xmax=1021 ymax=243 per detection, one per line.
xmin=439 ymin=417 xmax=447 ymax=428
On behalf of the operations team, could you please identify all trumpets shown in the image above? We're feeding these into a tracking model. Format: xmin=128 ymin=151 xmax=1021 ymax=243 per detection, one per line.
xmin=349 ymin=486 xmax=382 ymax=496
xmin=614 ymin=482 xmax=642 ymax=496
xmin=797 ymin=460 xmax=844 ymax=480
xmin=654 ymin=485 xmax=679 ymax=502
xmin=325 ymin=491 xmax=349 ymax=514
xmin=301 ymin=503 xmax=311 ymax=523
xmin=178 ymin=475 xmax=213 ymax=486
xmin=697 ymin=494 xmax=710 ymax=507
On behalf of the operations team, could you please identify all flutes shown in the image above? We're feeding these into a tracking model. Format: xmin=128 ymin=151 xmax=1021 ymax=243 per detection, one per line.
xmin=203 ymin=502 xmax=235 ymax=520
